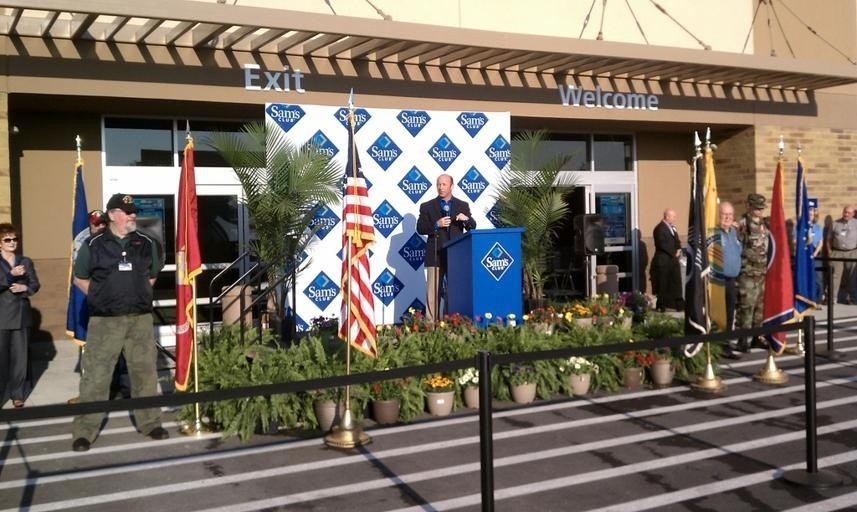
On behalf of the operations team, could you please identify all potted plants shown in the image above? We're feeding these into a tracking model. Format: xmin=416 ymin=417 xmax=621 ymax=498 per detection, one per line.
xmin=194 ymin=292 xmax=726 ymax=429
xmin=201 ymin=120 xmax=340 ymax=337
xmin=487 ymin=126 xmax=577 ymax=312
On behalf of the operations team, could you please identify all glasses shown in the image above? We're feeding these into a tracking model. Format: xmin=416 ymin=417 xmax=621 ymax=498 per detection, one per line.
xmin=3 ymin=237 xmax=18 ymax=243
xmin=124 ymin=209 xmax=137 ymax=215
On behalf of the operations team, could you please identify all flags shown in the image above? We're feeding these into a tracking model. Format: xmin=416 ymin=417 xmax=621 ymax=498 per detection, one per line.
xmin=678 ymin=141 xmax=736 ymax=360
xmin=337 ymin=108 xmax=378 ymax=359
xmin=65 ymin=155 xmax=90 ymax=347
xmin=789 ymin=155 xmax=821 ymax=324
xmin=173 ymin=136 xmax=204 ymax=394
xmin=762 ymin=153 xmax=794 ymax=360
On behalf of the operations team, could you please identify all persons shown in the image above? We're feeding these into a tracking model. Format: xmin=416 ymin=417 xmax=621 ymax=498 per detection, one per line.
xmin=649 ymin=207 xmax=686 ymax=313
xmin=68 ymin=207 xmax=130 ymax=404
xmin=73 ymin=191 xmax=170 ymax=451
xmin=0 ymin=221 xmax=41 ymax=407
xmin=793 ymin=205 xmax=826 ymax=305
xmin=417 ymin=174 xmax=476 ymax=328
xmin=730 ymin=192 xmax=771 ymax=352
xmin=823 ymin=203 xmax=857 ymax=305
xmin=709 ymin=201 xmax=742 ymax=359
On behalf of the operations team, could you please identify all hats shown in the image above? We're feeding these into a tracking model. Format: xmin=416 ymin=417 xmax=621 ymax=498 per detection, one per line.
xmin=89 ymin=209 xmax=108 ymax=226
xmin=749 ymin=194 xmax=768 ymax=210
xmin=107 ymin=192 xmax=143 ymax=211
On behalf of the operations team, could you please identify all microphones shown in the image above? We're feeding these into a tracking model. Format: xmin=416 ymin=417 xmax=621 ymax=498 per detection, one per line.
xmin=443 ymin=204 xmax=451 ymax=232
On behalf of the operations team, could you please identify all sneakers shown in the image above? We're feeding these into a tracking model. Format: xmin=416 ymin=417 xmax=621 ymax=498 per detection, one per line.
xmin=750 ymin=339 xmax=768 ymax=348
xmin=72 ymin=438 xmax=90 ymax=452
xmin=12 ymin=399 xmax=25 ymax=407
xmin=150 ymin=427 xmax=168 ymax=439
xmin=68 ymin=396 xmax=80 ymax=403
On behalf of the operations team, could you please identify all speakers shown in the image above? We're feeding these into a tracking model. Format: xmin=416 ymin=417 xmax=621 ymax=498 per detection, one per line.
xmin=573 ymin=213 xmax=606 ymax=255
xmin=136 ymin=217 xmax=165 ymax=268
